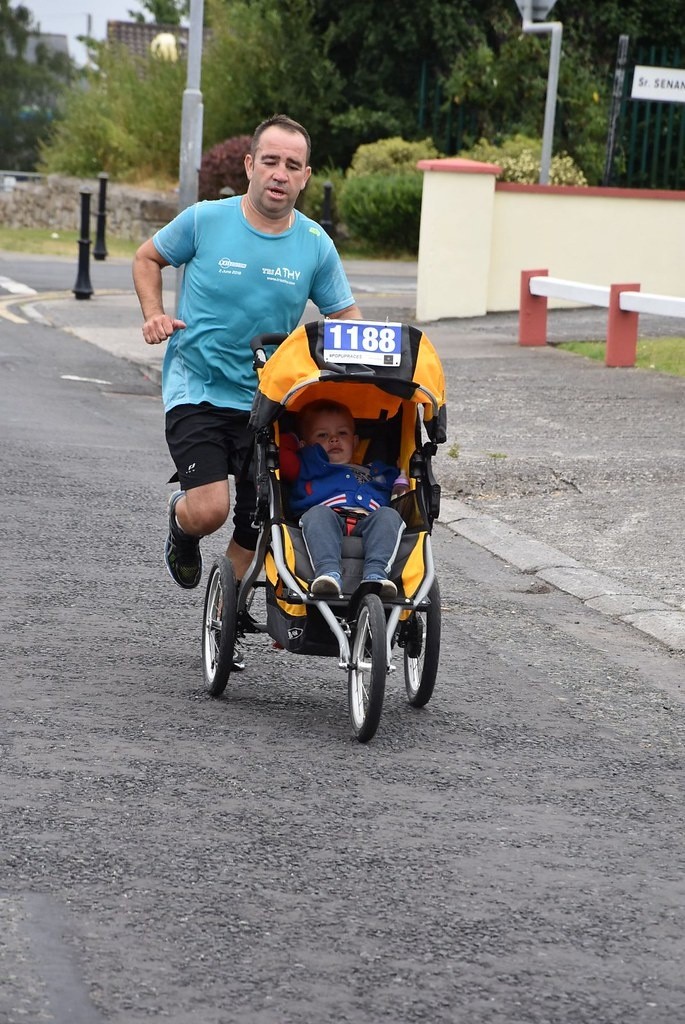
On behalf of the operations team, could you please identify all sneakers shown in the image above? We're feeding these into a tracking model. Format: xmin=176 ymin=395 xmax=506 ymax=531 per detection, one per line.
xmin=308 ymin=568 xmax=343 ymax=595
xmin=361 ymin=574 xmax=399 ymax=598
xmin=214 ymin=628 xmax=245 ymax=670
xmin=164 ymin=488 xmax=204 ymax=590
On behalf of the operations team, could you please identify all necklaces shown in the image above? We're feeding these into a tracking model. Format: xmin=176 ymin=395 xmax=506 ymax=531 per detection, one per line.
xmin=242 ymin=202 xmax=292 ymax=229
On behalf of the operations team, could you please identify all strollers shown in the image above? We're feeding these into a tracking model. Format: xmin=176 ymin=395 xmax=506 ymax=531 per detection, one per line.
xmin=201 ymin=319 xmax=441 ymax=743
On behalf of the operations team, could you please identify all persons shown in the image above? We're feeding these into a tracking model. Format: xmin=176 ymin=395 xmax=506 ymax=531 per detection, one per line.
xmin=284 ymin=398 xmax=406 ymax=599
xmin=132 ymin=116 xmax=362 ymax=633
